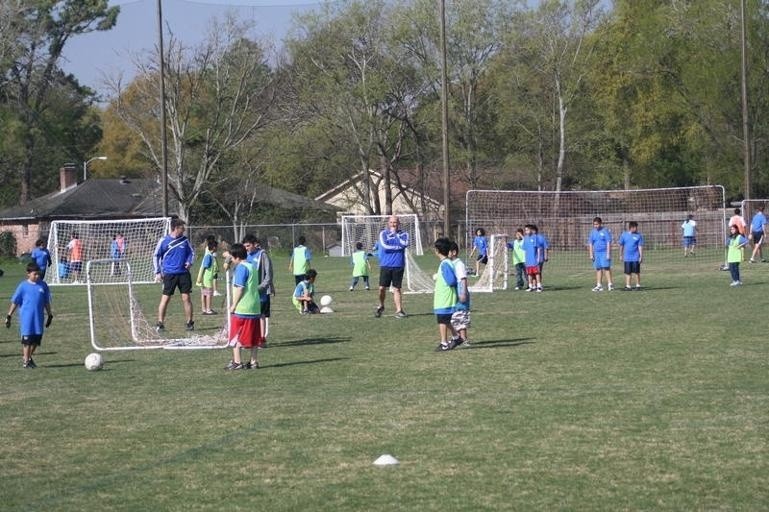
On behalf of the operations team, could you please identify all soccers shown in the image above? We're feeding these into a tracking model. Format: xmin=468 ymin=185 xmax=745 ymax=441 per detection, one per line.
xmin=320 ymin=294 xmax=333 ymax=306
xmin=84 ymin=352 xmax=103 ymax=371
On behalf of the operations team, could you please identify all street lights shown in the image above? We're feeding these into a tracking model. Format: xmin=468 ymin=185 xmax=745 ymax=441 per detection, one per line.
xmin=83 ymin=156 xmax=108 ymax=180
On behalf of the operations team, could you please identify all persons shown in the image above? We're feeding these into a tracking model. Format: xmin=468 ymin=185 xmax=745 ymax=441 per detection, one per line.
xmin=748 ymin=204 xmax=768 ymax=263
xmin=589 ymin=218 xmax=614 ymax=291
xmin=725 ymin=225 xmax=749 ymax=286
xmin=619 ymin=221 xmax=644 ymax=290
xmin=728 ymin=209 xmax=747 ymax=261
xmin=681 ymin=215 xmax=699 ymax=257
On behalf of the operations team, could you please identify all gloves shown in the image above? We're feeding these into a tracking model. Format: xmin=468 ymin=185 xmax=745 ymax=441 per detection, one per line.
xmin=46 ymin=316 xmax=53 ymax=327
xmin=5 ymin=314 xmax=12 ymax=329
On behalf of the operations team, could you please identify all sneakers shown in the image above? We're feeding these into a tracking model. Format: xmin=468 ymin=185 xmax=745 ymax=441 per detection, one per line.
xmin=374 ymin=305 xmax=385 ymax=318
xmin=448 ymin=336 xmax=471 ymax=347
xmin=185 ymin=321 xmax=195 ymax=331
xmin=223 ymin=360 xmax=243 ymax=371
xmin=302 ymin=310 xmax=316 ymax=315
xmin=749 ymin=257 xmax=767 ymax=264
xmin=256 ymin=337 xmax=266 ymax=348
xmin=394 ymin=310 xmax=410 ymax=320
xmin=592 ymin=284 xmax=642 ymax=293
xmin=514 ymin=285 xmax=545 ymax=293
xmin=22 ymin=359 xmax=37 ymax=370
xmin=202 ymin=310 xmax=218 ymax=315
xmin=156 ymin=321 xmax=166 ymax=331
xmin=729 ymin=280 xmax=744 ymax=288
xmin=212 ymin=290 xmax=224 ymax=297
xmin=349 ymin=286 xmax=372 ymax=291
xmin=433 ymin=343 xmax=453 ymax=352
xmin=244 ymin=360 xmax=260 ymax=369
